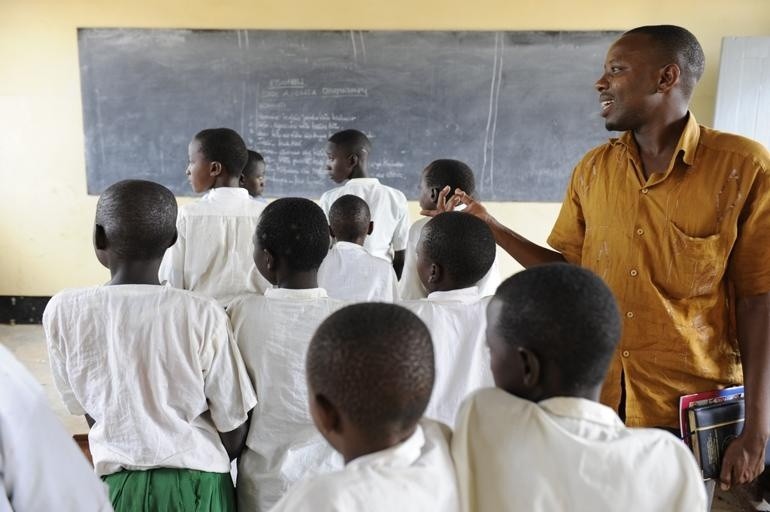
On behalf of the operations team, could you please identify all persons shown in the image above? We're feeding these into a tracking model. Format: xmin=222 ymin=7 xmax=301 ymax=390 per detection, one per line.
xmin=397 ymin=158 xmax=500 ymax=300
xmin=0 ymin=342 xmax=119 ymax=511
xmin=157 ymin=127 xmax=273 ymax=308
xmin=40 ymin=180 xmax=258 ymax=511
xmin=223 ymin=197 xmax=355 ymax=510
xmin=318 ymin=130 xmax=409 ymax=282
xmin=240 ymin=151 xmax=269 ymax=204
xmin=266 ymin=302 xmax=463 ymax=511
xmin=419 ymin=25 xmax=770 ymax=511
xmin=318 ymin=194 xmax=408 ymax=308
xmin=449 ymin=262 xmax=713 ymax=511
xmin=398 ymin=211 xmax=496 ymax=431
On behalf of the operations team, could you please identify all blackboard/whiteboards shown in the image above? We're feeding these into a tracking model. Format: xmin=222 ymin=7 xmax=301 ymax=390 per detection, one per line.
xmin=75 ymin=27 xmax=628 ymax=203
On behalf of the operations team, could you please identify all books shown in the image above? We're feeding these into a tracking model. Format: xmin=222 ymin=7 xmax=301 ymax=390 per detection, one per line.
xmin=687 ymin=398 xmax=770 ymax=482
xmin=680 ymin=384 xmax=745 ymax=451
